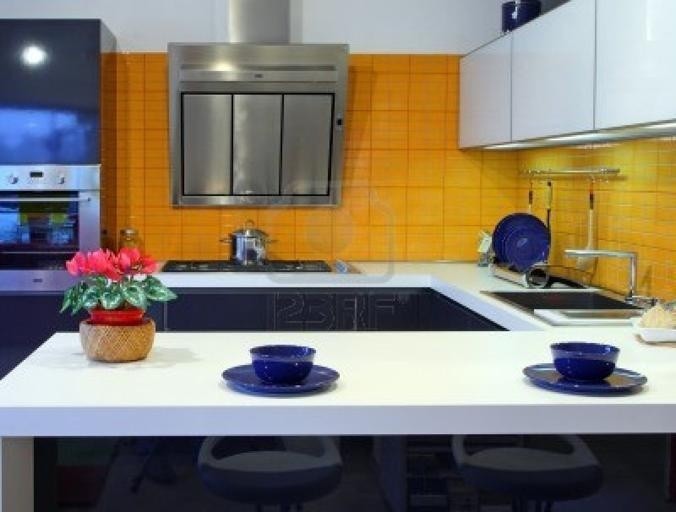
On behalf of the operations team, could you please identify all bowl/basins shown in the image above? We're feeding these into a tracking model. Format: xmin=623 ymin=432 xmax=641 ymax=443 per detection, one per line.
xmin=548 ymin=341 xmax=623 ymax=382
xmin=246 ymin=344 xmax=317 ymax=384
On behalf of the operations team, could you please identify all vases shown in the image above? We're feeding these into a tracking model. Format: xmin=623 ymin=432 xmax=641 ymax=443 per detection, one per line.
xmin=87 ymin=308 xmax=144 ymax=326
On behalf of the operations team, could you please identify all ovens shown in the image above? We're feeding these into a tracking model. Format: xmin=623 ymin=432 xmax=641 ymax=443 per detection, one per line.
xmin=1 ymin=164 xmax=104 ymax=294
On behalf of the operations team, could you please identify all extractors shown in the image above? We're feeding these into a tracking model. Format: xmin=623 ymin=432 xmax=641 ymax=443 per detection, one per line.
xmin=167 ymin=0 xmax=349 ymax=208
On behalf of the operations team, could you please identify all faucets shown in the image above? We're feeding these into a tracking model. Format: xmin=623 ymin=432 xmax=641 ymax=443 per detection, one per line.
xmin=563 ymin=247 xmax=638 ymax=295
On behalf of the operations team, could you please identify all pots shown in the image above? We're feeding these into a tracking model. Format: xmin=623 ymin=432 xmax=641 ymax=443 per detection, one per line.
xmin=218 ymin=218 xmax=280 ymax=263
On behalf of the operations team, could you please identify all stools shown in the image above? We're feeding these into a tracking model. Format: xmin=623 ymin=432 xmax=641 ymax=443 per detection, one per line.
xmin=197 ymin=436 xmax=344 ymax=512
xmin=451 ymin=435 xmax=603 ymax=512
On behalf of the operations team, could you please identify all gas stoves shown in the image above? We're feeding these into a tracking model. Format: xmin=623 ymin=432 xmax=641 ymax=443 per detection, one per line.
xmin=160 ymin=257 xmax=364 ymax=275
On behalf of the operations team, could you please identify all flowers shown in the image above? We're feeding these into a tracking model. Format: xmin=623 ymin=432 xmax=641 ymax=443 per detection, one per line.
xmin=58 ymin=249 xmax=179 ymax=316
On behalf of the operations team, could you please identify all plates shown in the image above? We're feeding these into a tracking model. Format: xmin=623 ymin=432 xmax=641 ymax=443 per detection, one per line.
xmin=631 ymin=316 xmax=676 ymax=344
xmin=220 ymin=364 xmax=342 ymax=396
xmin=494 ymin=211 xmax=550 ymax=272
xmin=520 ymin=361 xmax=649 ymax=397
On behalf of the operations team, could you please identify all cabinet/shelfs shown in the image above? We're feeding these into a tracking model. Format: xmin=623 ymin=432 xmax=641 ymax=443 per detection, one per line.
xmin=166 ymin=289 xmax=369 ymax=330
xmin=370 ymin=289 xmax=430 ymax=331
xmin=456 ymin=0 xmax=676 ymax=151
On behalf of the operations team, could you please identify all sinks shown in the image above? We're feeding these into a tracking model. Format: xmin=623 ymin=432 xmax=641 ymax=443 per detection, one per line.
xmin=480 ymin=289 xmax=652 ymax=325
